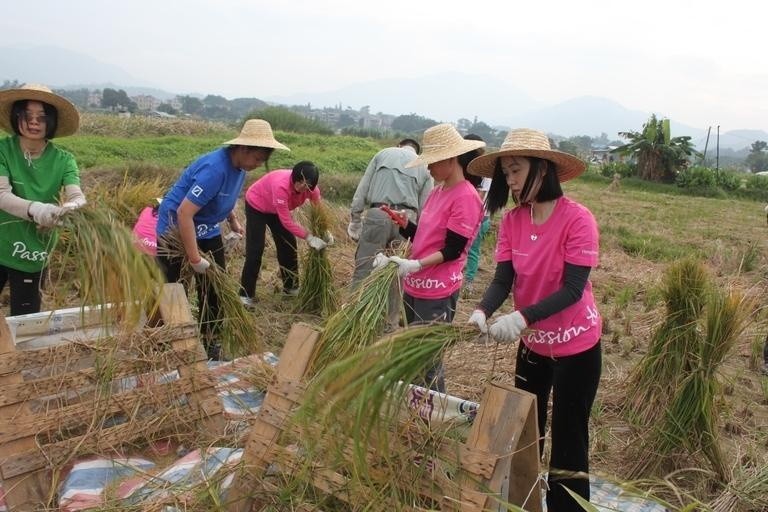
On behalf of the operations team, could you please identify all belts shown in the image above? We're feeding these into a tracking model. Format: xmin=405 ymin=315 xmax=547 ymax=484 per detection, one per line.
xmin=370 ymin=203 xmax=418 ymax=213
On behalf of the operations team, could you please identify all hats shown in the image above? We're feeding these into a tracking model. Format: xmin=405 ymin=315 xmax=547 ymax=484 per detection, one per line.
xmin=466 ymin=128 xmax=586 ymax=183
xmin=222 ymin=119 xmax=291 ymax=152
xmin=1 ymin=84 xmax=79 ymax=138
xmin=404 ymin=124 xmax=486 ymax=168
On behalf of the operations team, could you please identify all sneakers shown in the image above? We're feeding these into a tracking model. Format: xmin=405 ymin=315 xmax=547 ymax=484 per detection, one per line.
xmin=283 ymin=288 xmax=301 ymax=295
xmin=240 ymin=295 xmax=256 ymax=307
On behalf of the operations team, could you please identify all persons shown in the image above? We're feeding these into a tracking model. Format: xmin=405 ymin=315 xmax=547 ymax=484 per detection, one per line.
xmin=347 ymin=139 xmax=435 ymax=334
xmin=462 ymin=133 xmax=493 ymax=290
xmin=237 ymin=160 xmax=333 ymax=310
xmin=0 ymin=101 xmax=87 ymax=316
xmin=129 ymin=204 xmax=161 ymax=259
xmin=464 ymin=155 xmax=603 ymax=512
xmin=156 ymin=145 xmax=274 ymax=349
xmin=376 ymin=150 xmax=484 ymax=392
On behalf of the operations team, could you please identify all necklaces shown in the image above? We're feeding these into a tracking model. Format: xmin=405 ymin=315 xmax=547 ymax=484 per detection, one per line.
xmin=530 ymin=199 xmax=556 ymax=240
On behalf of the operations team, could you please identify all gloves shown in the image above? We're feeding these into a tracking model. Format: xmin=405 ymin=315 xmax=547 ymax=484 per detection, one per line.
xmin=489 ymin=310 xmax=527 ymax=344
xmin=347 ymin=220 xmax=362 ymax=241
xmin=373 ymin=253 xmax=421 ymax=278
xmin=224 ymin=232 xmax=241 ymax=249
xmin=189 ymin=257 xmax=210 ymax=274
xmin=306 ymin=236 xmax=326 ymax=252
xmin=29 ymin=202 xmax=64 ymax=228
xmin=59 ymin=199 xmax=86 ymax=217
xmin=465 ymin=310 xmax=487 ymax=344
xmin=322 ymin=230 xmax=333 ymax=245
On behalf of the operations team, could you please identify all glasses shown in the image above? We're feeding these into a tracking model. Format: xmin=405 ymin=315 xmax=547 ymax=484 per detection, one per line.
xmin=17 ymin=113 xmax=48 ymax=124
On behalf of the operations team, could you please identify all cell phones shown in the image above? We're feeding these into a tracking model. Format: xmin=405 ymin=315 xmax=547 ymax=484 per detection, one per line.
xmin=379 ymin=205 xmax=404 ymax=226
